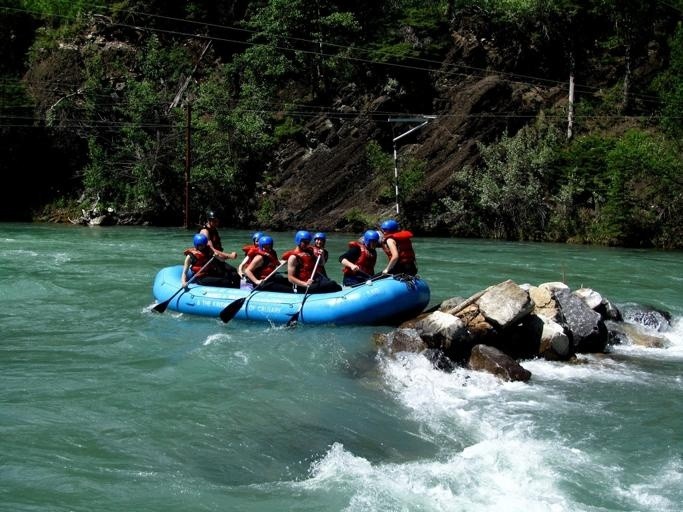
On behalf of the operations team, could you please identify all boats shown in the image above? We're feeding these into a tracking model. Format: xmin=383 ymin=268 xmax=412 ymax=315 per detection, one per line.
xmin=152 ymin=264 xmax=429 ymax=329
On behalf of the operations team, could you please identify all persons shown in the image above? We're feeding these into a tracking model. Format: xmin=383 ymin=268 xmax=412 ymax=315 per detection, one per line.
xmin=237 ymin=231 xmax=264 ymax=279
xmin=374 ymin=220 xmax=418 ymax=278
xmin=282 ymin=231 xmax=342 ymax=294
xmin=311 ymin=232 xmax=329 ymax=268
xmin=245 ymin=236 xmax=291 ymax=293
xmin=199 ymin=210 xmax=238 ymax=262
xmin=339 ymin=229 xmax=379 ymax=288
xmin=181 ymin=233 xmax=240 ymax=289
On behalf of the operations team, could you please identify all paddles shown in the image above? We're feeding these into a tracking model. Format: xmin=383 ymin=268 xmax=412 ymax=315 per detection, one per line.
xmin=151 ymin=257 xmax=215 ymax=314
xmin=220 ymin=261 xmax=285 ymax=323
xmin=286 ymin=254 xmax=321 ymax=331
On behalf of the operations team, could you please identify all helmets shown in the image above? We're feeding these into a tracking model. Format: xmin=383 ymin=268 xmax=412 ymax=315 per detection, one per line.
xmin=252 ymin=232 xmax=264 ymax=241
xmin=294 ymin=230 xmax=312 ymax=246
xmin=380 ymin=220 xmax=398 ymax=232
xmin=313 ymin=232 xmax=326 ymax=240
xmin=193 ymin=234 xmax=207 ymax=248
xmin=258 ymin=235 xmax=273 ymax=250
xmin=205 ymin=210 xmax=217 ymax=219
xmin=363 ymin=229 xmax=379 ymax=248
